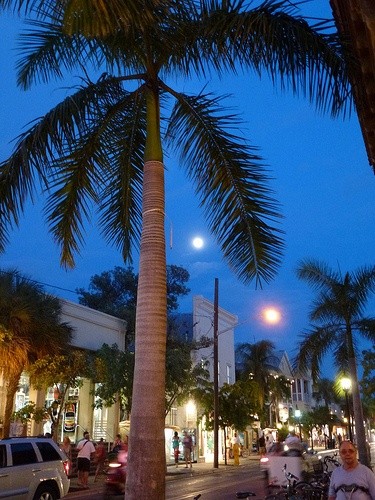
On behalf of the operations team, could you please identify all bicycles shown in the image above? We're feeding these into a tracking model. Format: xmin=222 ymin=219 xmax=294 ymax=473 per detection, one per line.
xmin=191 ymin=452 xmax=373 ymax=500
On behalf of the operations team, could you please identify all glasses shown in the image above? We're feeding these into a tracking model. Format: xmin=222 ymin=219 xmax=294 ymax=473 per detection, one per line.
xmin=339 ymin=449 xmax=356 ymax=455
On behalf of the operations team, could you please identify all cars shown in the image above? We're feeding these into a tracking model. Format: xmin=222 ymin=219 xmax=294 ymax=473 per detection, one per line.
xmin=260 ymin=441 xmax=322 ymax=477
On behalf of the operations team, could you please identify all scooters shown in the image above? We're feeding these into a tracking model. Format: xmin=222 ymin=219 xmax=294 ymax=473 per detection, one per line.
xmin=104 ymin=461 xmax=128 ymax=495
xmin=229 ymin=443 xmax=249 ymax=458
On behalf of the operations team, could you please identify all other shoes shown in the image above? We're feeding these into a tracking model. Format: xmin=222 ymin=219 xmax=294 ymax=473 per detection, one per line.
xmin=80 ymin=486 xmax=89 ymax=490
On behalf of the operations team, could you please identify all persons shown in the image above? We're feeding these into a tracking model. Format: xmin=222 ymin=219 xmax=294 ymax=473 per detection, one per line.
xmin=172 ymin=430 xmax=275 ymax=468
xmin=46 ymin=432 xmax=128 ymax=489
xmin=328 ymin=441 xmax=375 ymax=500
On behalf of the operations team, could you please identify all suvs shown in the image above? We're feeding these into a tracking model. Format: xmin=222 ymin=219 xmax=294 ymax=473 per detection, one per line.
xmin=0 ymin=434 xmax=73 ymax=500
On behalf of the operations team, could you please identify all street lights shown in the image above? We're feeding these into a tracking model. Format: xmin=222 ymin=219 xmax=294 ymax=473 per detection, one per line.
xmin=294 ymin=407 xmax=302 ymax=437
xmin=340 ymin=371 xmax=353 ymax=443
xmin=185 ymin=402 xmax=197 ymax=468
xmin=212 ymin=277 xmax=282 ymax=468
